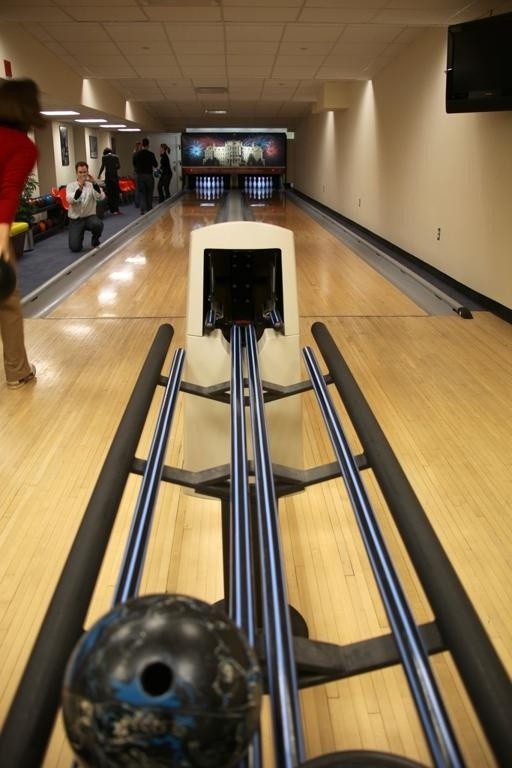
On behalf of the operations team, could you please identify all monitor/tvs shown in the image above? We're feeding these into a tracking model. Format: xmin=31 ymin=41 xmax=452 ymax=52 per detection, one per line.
xmin=446 ymin=12 xmax=512 ymax=114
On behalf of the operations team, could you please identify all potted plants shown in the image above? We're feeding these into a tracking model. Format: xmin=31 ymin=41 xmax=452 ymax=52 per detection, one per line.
xmin=14 ymin=171 xmax=41 ymax=252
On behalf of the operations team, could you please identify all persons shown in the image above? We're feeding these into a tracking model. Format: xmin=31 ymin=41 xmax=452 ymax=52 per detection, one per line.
xmin=157 ymin=144 xmax=172 ymax=203
xmin=97 ymin=147 xmax=123 ymax=215
xmin=132 ymin=143 xmax=142 ymax=208
xmin=65 ymin=161 xmax=106 ymax=252
xmin=132 ymin=138 xmax=158 ymax=216
xmin=0 ymin=77 xmax=57 ymax=389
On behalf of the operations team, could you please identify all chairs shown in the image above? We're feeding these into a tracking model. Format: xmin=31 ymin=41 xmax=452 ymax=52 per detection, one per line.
xmin=52 ymin=176 xmax=135 ymax=220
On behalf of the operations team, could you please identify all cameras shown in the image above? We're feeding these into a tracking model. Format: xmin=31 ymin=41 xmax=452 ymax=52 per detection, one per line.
xmin=81 ymin=173 xmax=87 ymax=180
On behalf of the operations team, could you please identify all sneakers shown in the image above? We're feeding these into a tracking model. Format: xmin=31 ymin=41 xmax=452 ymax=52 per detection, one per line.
xmin=6 ymin=363 xmax=36 ymax=391
